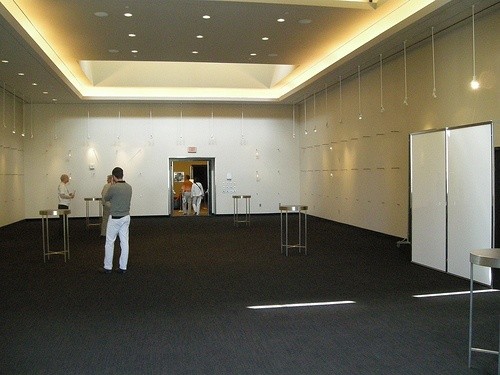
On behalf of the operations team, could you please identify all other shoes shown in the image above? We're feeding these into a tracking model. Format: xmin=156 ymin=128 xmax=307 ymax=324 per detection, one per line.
xmin=99 ymin=268 xmax=125 ymax=274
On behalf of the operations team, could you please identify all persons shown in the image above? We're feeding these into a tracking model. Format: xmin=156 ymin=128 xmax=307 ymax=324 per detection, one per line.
xmin=182 ymin=174 xmax=195 ymax=215
xmin=191 ymin=176 xmax=205 ymax=216
xmin=58 ymin=174 xmax=76 ymax=237
xmin=102 ymin=174 xmax=116 ymax=237
xmin=100 ymin=166 xmax=133 ymax=274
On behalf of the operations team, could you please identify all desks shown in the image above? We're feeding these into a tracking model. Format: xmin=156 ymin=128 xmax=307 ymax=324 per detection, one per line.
xmin=467 ymin=248 xmax=500 ymax=369
xmin=84 ymin=196 xmax=104 ymax=231
xmin=232 ymin=194 xmax=251 ymax=227
xmin=279 ymin=204 xmax=310 ymax=254
xmin=38 ymin=207 xmax=72 ymax=263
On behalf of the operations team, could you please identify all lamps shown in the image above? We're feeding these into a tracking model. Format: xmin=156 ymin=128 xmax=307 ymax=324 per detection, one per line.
xmin=2 ymin=4 xmax=480 ymax=143
